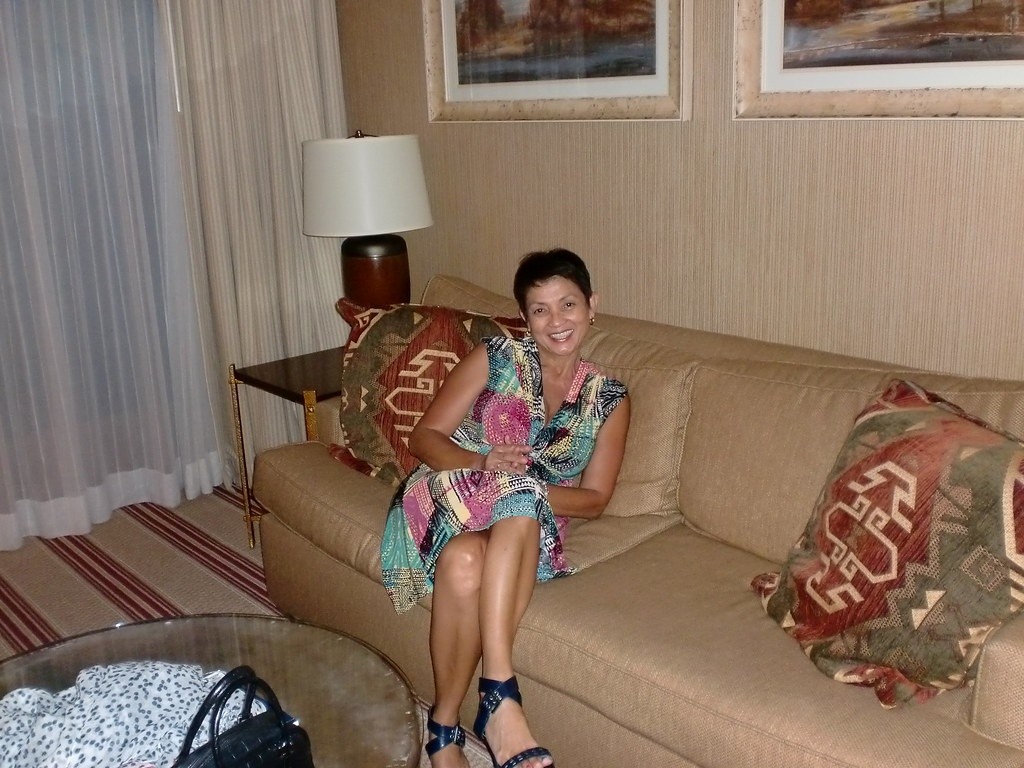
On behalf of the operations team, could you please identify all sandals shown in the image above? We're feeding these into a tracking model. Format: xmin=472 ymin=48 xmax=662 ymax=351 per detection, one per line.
xmin=474 ymin=676 xmax=555 ymax=768
xmin=425 ymin=707 xmax=465 ymax=756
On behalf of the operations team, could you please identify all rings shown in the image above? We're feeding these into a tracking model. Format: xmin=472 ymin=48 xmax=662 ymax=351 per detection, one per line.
xmin=497 ymin=461 xmax=503 ymax=471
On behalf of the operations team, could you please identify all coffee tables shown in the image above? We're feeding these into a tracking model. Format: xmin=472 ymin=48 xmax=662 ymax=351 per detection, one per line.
xmin=0 ymin=612 xmax=422 ymax=768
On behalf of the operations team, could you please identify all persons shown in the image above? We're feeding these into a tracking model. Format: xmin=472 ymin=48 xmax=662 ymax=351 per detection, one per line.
xmin=380 ymin=247 xmax=632 ymax=768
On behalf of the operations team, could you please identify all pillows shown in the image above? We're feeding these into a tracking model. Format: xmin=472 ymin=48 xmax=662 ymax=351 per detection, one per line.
xmin=326 ymin=299 xmax=528 ymax=489
xmin=749 ymin=373 xmax=1024 ymax=709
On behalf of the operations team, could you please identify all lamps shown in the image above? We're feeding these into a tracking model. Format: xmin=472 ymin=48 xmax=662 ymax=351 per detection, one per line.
xmin=302 ymin=130 xmax=434 ymax=313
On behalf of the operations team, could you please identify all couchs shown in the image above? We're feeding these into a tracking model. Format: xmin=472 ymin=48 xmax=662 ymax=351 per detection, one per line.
xmin=249 ymin=273 xmax=1024 ymax=768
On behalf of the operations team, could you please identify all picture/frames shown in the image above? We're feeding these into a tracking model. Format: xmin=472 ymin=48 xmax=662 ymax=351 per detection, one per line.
xmin=731 ymin=0 xmax=1024 ymax=120
xmin=421 ymin=1 xmax=693 ymax=122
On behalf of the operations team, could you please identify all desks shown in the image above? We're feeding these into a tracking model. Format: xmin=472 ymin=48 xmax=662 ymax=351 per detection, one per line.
xmin=229 ymin=344 xmax=345 ymax=548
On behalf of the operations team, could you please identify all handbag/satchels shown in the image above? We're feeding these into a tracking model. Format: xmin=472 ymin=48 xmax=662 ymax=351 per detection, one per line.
xmin=169 ymin=666 xmax=315 ymax=768
